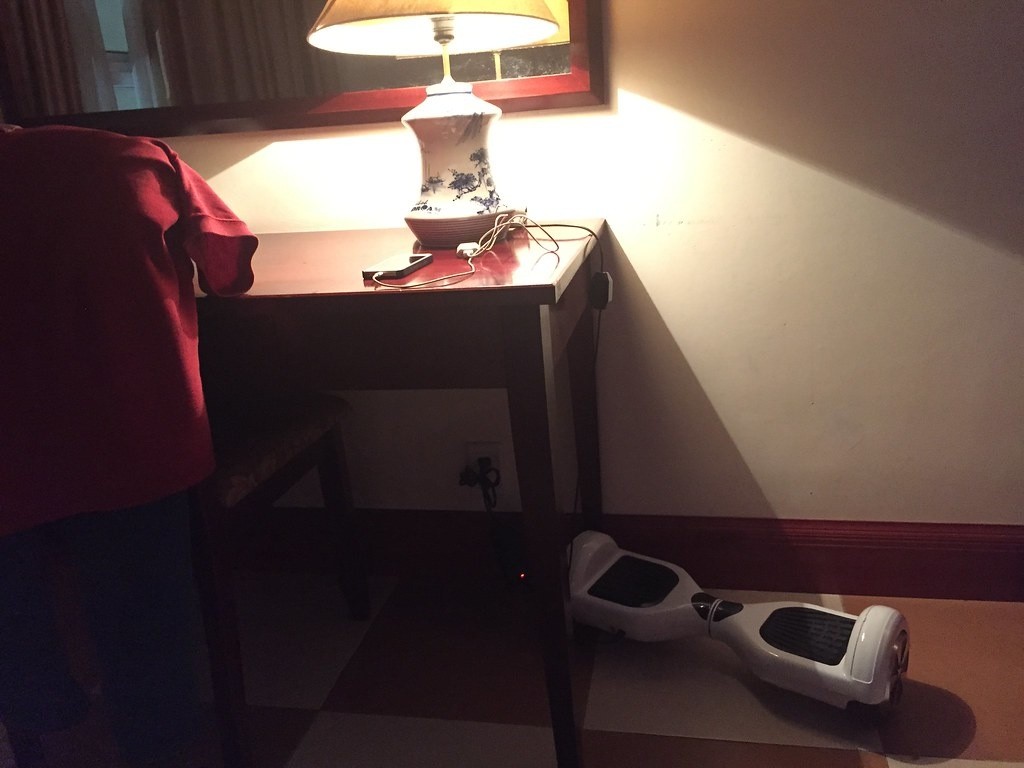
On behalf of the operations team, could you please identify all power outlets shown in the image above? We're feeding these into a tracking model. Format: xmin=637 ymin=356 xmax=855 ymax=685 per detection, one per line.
xmin=465 ymin=440 xmax=505 ymax=496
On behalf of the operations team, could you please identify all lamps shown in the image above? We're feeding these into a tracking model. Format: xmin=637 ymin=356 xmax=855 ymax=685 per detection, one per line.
xmin=305 ymin=0 xmax=560 ymax=250
xmin=396 ymin=0 xmax=570 ymax=80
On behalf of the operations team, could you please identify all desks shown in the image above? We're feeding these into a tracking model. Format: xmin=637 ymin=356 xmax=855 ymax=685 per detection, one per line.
xmin=194 ymin=218 xmax=606 ymax=768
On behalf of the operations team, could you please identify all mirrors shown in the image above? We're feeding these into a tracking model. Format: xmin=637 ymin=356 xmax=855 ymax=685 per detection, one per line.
xmin=0 ymin=0 xmax=604 ymax=138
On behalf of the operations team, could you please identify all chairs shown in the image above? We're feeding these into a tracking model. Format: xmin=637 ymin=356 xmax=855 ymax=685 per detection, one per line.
xmin=0 ymin=124 xmax=372 ymax=768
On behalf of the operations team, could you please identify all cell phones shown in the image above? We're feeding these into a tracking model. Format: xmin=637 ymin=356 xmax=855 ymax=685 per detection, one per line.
xmin=362 ymin=253 xmax=433 ymax=280
